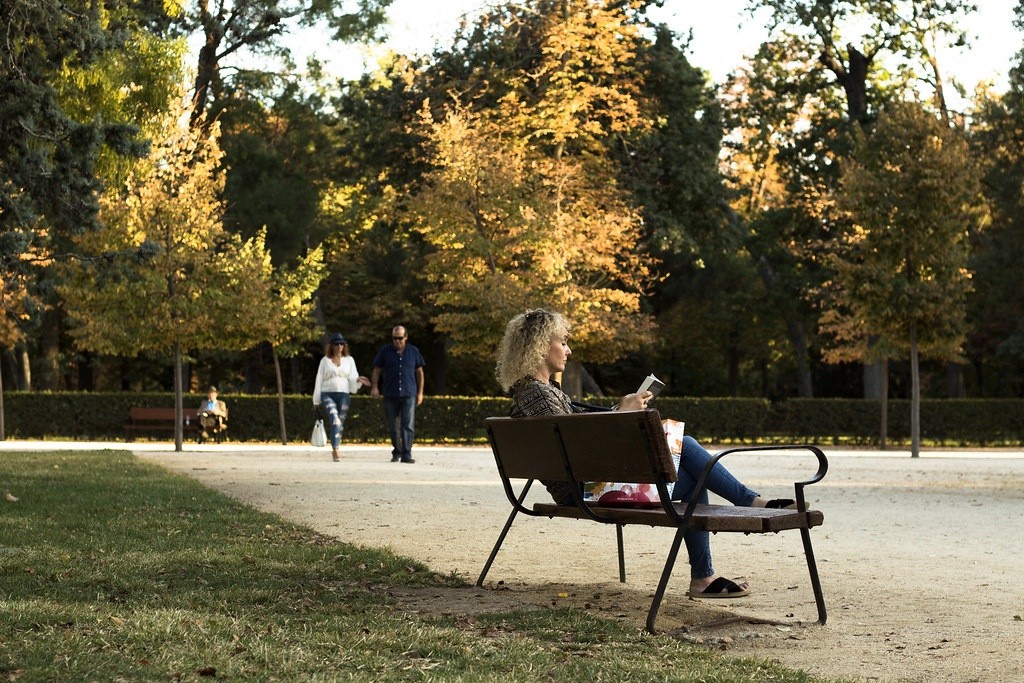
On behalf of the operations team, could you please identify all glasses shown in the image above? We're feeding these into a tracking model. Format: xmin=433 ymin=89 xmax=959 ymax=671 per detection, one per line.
xmin=333 ymin=342 xmax=344 ymax=346
xmin=392 ymin=336 xmax=403 ymax=340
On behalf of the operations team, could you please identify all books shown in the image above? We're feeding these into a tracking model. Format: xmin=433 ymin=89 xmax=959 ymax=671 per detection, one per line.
xmin=636 ymin=371 xmax=665 ymax=412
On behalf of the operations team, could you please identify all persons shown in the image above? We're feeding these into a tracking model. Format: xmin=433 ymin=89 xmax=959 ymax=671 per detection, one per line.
xmin=497 ymin=310 xmax=811 ymax=599
xmin=197 ymin=385 xmax=226 ymax=444
xmin=312 ymin=332 xmax=371 ymax=462
xmin=371 ymin=325 xmax=425 ymax=464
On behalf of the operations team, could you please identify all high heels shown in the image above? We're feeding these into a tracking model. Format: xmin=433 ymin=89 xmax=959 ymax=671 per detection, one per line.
xmin=331 ymin=451 xmax=340 ymax=462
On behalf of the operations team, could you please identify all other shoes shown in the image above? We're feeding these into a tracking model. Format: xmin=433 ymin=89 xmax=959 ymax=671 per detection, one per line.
xmin=401 ymin=456 xmax=415 ymax=463
xmin=391 ymin=454 xmax=400 ymax=462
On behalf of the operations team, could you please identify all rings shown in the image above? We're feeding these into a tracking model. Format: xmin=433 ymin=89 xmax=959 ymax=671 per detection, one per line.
xmin=644 ymin=398 xmax=647 ymax=405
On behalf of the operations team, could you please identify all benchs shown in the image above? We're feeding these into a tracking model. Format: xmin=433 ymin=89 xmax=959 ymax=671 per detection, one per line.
xmin=123 ymin=406 xmax=227 ymax=444
xmin=475 ymin=407 xmax=830 ymax=633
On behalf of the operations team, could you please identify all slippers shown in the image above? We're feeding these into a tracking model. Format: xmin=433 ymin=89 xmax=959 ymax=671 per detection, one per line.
xmin=689 ymin=576 xmax=750 ymax=598
xmin=765 ymin=499 xmax=810 ymax=511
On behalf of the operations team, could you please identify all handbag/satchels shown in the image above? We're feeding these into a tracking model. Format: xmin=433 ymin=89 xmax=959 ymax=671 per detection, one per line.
xmin=583 ymin=418 xmax=686 ymax=503
xmin=310 ymin=419 xmax=327 ymax=447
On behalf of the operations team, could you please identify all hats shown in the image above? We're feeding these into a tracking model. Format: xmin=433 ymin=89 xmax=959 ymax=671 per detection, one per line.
xmin=207 ymin=386 xmax=219 ymax=394
xmin=330 ymin=333 xmax=346 ymax=343
